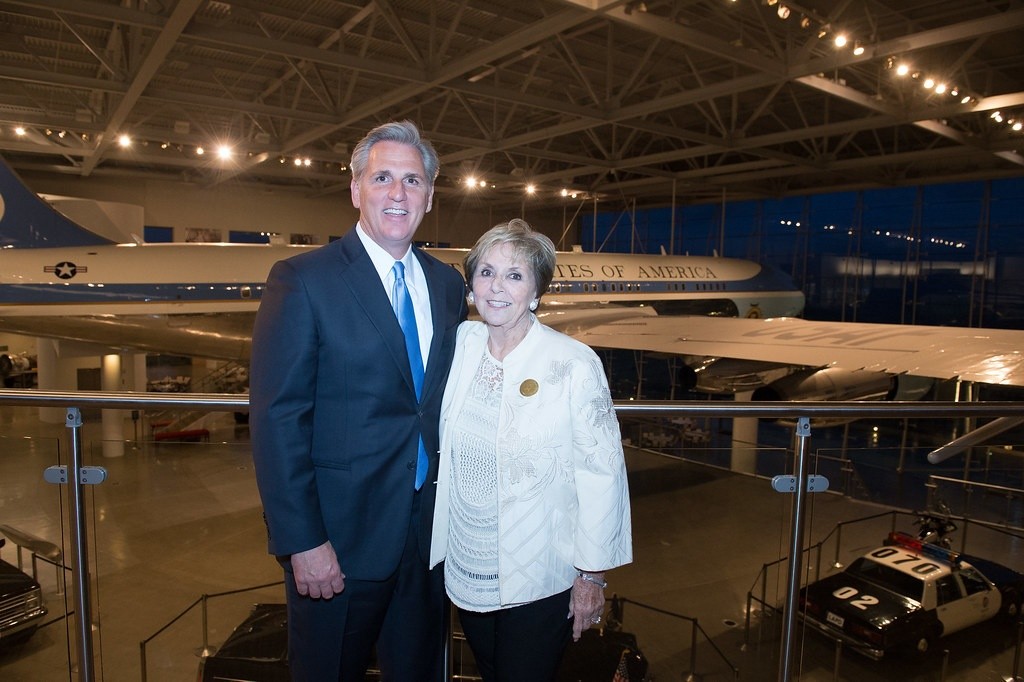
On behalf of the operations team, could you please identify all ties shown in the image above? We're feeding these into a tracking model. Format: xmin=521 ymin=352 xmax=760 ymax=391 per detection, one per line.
xmin=391 ymin=261 xmax=430 ymax=490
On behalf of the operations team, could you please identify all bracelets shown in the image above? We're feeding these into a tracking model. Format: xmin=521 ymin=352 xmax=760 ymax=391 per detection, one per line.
xmin=580 ymin=573 xmax=608 ymax=589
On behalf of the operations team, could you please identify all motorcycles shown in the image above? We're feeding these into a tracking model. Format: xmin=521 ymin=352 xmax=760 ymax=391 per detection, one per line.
xmin=910 ymin=491 xmax=956 ymax=548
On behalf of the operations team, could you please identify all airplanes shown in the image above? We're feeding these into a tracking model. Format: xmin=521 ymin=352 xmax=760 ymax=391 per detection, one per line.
xmin=0 ymin=163 xmax=1024 ymax=463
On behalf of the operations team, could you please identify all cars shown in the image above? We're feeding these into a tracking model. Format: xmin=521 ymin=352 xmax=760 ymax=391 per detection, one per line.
xmin=1 ymin=536 xmax=49 ymax=652
xmin=793 ymin=531 xmax=1023 ymax=670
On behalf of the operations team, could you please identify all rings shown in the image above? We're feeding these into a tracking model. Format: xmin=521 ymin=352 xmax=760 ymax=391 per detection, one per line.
xmin=590 ymin=616 xmax=602 ymax=625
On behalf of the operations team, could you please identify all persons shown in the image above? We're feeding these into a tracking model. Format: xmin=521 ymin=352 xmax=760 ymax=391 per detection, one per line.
xmin=246 ymin=118 xmax=468 ymax=682
xmin=427 ymin=218 xmax=633 ymax=682
